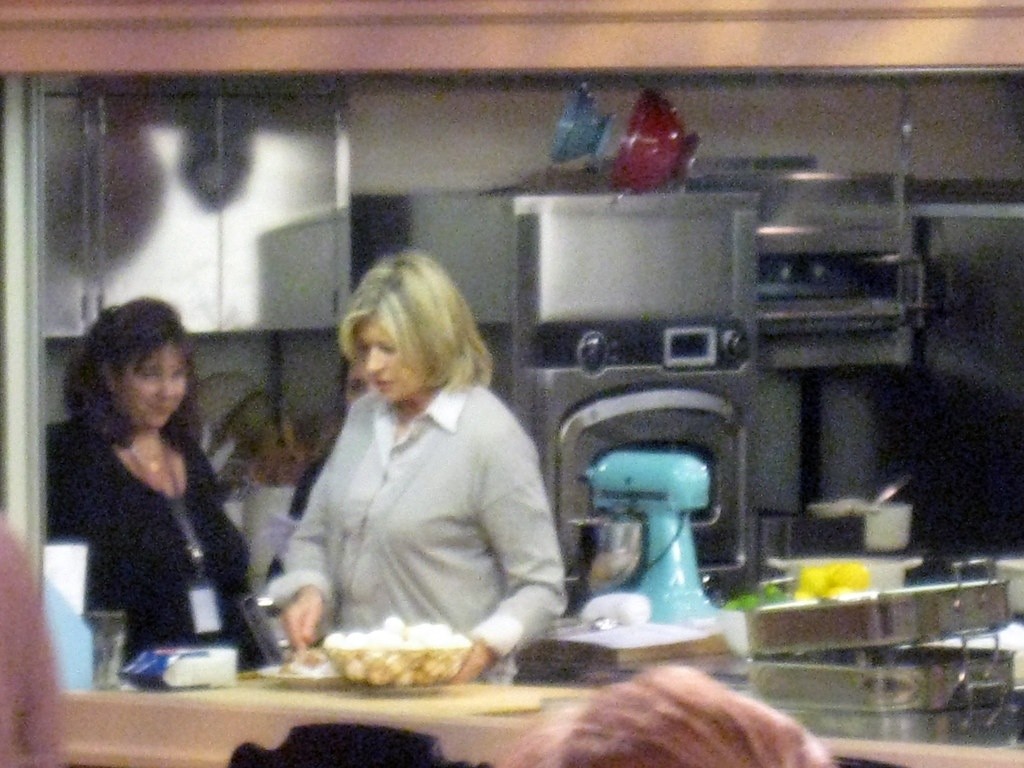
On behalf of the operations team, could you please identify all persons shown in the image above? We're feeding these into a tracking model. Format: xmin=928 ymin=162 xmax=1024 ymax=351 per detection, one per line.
xmin=268 ymin=252 xmax=569 ymax=688
xmin=522 ymin=658 xmax=836 ymax=768
xmin=45 ymin=297 xmax=274 ymax=672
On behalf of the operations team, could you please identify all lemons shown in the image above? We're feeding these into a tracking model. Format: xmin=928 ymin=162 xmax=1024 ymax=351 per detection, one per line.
xmin=725 ymin=564 xmax=870 ymax=611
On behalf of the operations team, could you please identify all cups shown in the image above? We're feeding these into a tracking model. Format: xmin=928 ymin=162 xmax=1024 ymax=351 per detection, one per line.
xmin=86 ymin=613 xmax=126 ymax=693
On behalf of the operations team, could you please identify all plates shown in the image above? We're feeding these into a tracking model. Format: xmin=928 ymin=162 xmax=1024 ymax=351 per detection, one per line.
xmin=257 ymin=666 xmax=337 ymax=684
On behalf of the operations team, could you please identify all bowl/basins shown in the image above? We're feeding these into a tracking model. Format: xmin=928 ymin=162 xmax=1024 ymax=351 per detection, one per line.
xmin=567 ymin=519 xmax=643 ymax=600
xmin=804 ymin=496 xmax=914 ymax=554
xmin=762 ymin=553 xmax=930 ymax=595
xmin=607 ymin=88 xmax=706 ymax=193
xmin=547 ymin=78 xmax=615 ymax=163
xmin=997 ymin=560 xmax=1024 ymax=617
xmin=326 ymin=644 xmax=473 ymax=687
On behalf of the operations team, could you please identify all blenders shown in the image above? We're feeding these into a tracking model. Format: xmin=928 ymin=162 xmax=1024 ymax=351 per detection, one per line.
xmin=575 ymin=448 xmax=718 ymax=630
